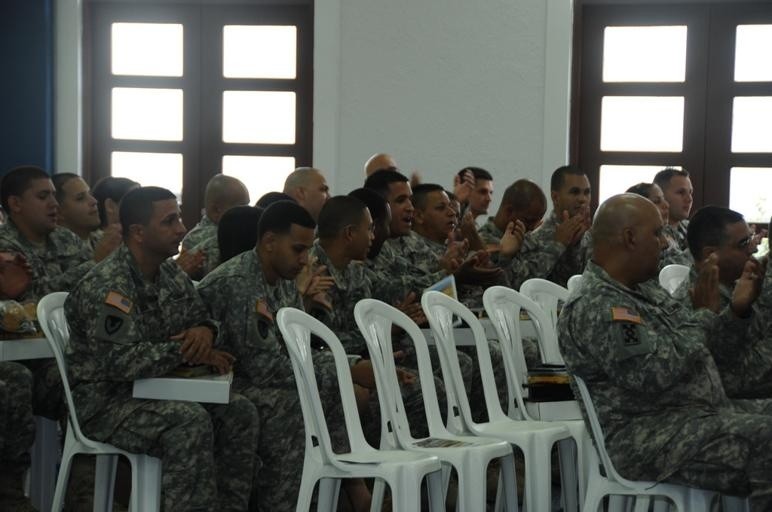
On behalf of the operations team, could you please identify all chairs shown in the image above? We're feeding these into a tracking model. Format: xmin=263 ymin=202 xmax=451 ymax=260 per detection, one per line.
xmin=520 ymin=278 xmax=570 ymax=366
xmin=574 ymin=374 xmax=750 ymax=512
xmin=37 ymin=291 xmax=161 ymax=512
xmin=567 ymin=275 xmax=582 ymax=288
xmin=659 ymin=264 xmax=691 ymax=295
xmin=354 ymin=299 xmax=519 ymax=511
xmin=277 ymin=307 xmax=444 ymax=512
xmin=421 ymin=290 xmax=577 ymax=512
xmin=483 ymin=286 xmax=604 ymax=512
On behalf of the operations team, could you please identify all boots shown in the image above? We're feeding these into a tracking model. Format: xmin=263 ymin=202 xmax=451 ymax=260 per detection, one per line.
xmin=0 ymin=474 xmax=41 ymax=512
xmin=67 ymin=466 xmax=127 ymax=512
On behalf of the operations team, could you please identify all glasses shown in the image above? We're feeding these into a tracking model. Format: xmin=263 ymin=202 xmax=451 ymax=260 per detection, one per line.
xmin=710 ymin=233 xmax=756 ymax=250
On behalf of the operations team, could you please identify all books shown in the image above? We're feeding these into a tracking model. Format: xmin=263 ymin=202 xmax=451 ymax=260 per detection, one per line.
xmin=132 ymin=366 xmax=237 ymax=405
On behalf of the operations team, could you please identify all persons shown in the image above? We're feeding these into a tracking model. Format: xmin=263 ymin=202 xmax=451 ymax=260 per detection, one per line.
xmin=686 ymin=208 xmax=772 ymax=393
xmin=195 ymin=195 xmax=319 ymax=509
xmin=255 ymin=193 xmax=304 ymax=209
xmin=412 ymin=184 xmax=513 ymax=423
xmin=281 ymin=167 xmax=331 ymax=223
xmin=627 ymin=183 xmax=682 ymax=254
xmin=305 ymin=196 xmax=438 ymax=504
xmin=651 ymin=166 xmax=692 ymax=228
xmin=181 ymin=174 xmax=248 ymax=253
xmin=62 ymin=186 xmax=259 ymax=511
xmin=1 ymin=166 xmax=122 ymax=508
xmin=92 ymin=176 xmax=142 ymax=231
xmin=457 ymin=167 xmax=491 ymax=233
xmin=447 ymin=192 xmax=524 ymax=310
xmin=519 ymin=165 xmax=595 ymax=282
xmin=53 ymin=171 xmax=121 ymax=256
xmin=363 ymin=169 xmax=479 ymax=434
xmin=348 ymin=190 xmax=443 ymax=432
xmin=476 ymin=179 xmax=564 ymax=283
xmin=186 ymin=204 xmax=261 ymax=278
xmin=555 ymin=194 xmax=770 ymax=509
xmin=363 ymin=155 xmax=398 ymax=174
